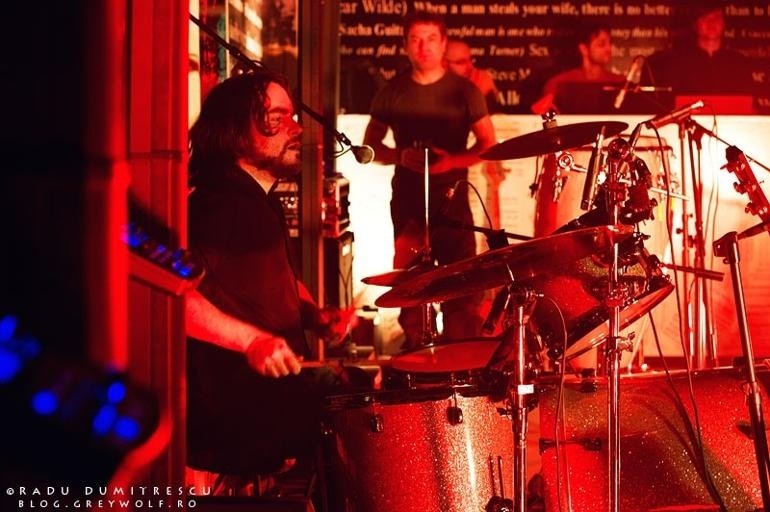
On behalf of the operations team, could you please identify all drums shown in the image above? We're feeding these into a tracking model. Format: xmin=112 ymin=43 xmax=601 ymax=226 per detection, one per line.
xmin=516 ymin=206 xmax=674 ymax=374
xmin=319 ymin=338 xmax=517 ymax=512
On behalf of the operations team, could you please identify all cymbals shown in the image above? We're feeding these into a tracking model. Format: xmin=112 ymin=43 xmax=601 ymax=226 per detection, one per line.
xmin=360 ymin=258 xmax=440 ymax=288
xmin=479 ymin=121 xmax=629 ymax=160
xmin=375 ymin=225 xmax=636 ymax=310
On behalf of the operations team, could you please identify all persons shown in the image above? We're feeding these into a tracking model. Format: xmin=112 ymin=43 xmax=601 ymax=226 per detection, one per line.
xmin=529 ymin=21 xmax=641 ymax=114
xmin=639 ymin=1 xmax=754 ymax=109
xmin=362 ymin=14 xmax=497 ymax=349
xmin=441 ymin=40 xmax=505 ymax=112
xmin=188 ymin=76 xmax=373 ymax=512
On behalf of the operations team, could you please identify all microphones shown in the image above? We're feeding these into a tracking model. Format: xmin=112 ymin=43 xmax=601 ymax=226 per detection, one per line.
xmin=644 ymin=101 xmax=707 ymax=129
xmin=614 ymin=53 xmax=645 ymax=111
xmin=335 ymin=132 xmax=376 ymax=165
